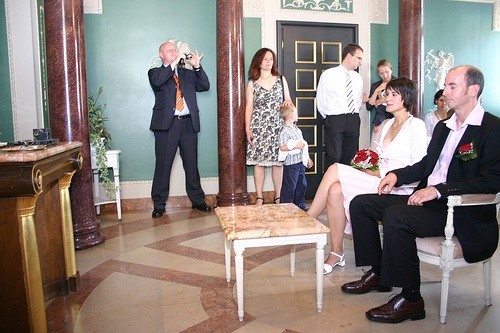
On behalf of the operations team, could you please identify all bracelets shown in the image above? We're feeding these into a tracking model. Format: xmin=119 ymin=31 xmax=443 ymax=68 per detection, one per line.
xmin=194 ymin=67 xmax=201 ymax=70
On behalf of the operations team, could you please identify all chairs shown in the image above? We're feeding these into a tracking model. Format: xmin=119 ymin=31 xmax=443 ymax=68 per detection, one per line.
xmin=378 ymin=193 xmax=500 ymax=324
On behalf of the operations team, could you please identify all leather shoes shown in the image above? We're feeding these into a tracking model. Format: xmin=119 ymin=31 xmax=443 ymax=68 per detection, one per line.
xmin=366 ymin=294 xmax=425 ymax=323
xmin=341 ymin=270 xmax=391 ymax=293
xmin=192 ymin=203 xmax=211 ymax=212
xmin=152 ymin=209 xmax=165 ymax=218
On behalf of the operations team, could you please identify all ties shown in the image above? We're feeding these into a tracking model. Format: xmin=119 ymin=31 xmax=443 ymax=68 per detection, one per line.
xmin=173 ymin=72 xmax=185 ymax=111
xmin=345 ymin=72 xmax=356 ymax=114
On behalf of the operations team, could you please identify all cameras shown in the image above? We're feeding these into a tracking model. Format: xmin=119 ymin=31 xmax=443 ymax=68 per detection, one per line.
xmin=184 ymin=52 xmax=194 ymax=60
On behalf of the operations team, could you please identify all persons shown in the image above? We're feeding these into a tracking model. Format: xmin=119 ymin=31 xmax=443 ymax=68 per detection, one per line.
xmin=317 ymin=43 xmax=364 ymax=173
xmin=428 ymin=50 xmax=455 ymax=89
xmin=342 ymin=64 xmax=500 ymax=323
xmin=307 ymin=77 xmax=428 ymax=275
xmin=365 ymin=59 xmax=398 ymax=127
xmin=148 ymin=43 xmax=211 ymax=218
xmin=278 ymin=105 xmax=313 ymax=212
xmin=424 ymin=89 xmax=452 ymax=138
xmin=244 ymin=48 xmax=293 ymax=205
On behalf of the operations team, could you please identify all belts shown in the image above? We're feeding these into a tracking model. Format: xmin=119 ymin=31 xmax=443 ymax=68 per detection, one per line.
xmin=171 ymin=113 xmax=190 ymax=120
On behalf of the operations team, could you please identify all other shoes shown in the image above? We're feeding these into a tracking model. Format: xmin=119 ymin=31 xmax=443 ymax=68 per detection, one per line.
xmin=273 ymin=196 xmax=280 ymax=204
xmin=256 ymin=196 xmax=264 ymax=205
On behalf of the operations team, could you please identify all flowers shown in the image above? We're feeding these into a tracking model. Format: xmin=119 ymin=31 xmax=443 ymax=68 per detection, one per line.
xmin=454 ymin=143 xmax=477 ymax=161
xmin=350 ymin=149 xmax=379 ymax=172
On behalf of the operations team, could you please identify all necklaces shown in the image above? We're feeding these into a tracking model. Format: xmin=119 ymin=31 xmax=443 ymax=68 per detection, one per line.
xmin=436 ymin=111 xmax=443 ymax=121
xmin=259 ymin=74 xmax=272 ymax=83
xmin=392 ymin=129 xmax=398 ymax=134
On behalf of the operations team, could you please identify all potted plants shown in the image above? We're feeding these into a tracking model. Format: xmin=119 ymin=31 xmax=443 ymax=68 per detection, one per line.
xmin=88 ymin=86 xmax=121 ymax=202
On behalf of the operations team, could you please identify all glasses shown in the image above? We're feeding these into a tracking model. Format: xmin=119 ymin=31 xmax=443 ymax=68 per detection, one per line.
xmin=351 ymin=53 xmax=361 ymax=61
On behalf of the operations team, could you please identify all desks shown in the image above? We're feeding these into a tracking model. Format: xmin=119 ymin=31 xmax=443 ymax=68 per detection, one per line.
xmin=91 ymin=150 xmax=122 ymax=219
xmin=0 ymin=141 xmax=84 ymax=333
xmin=214 ymin=202 xmax=330 ymax=321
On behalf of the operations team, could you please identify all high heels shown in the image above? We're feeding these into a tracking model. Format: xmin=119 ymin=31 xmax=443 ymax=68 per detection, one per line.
xmin=322 ymin=250 xmax=346 ymax=275
